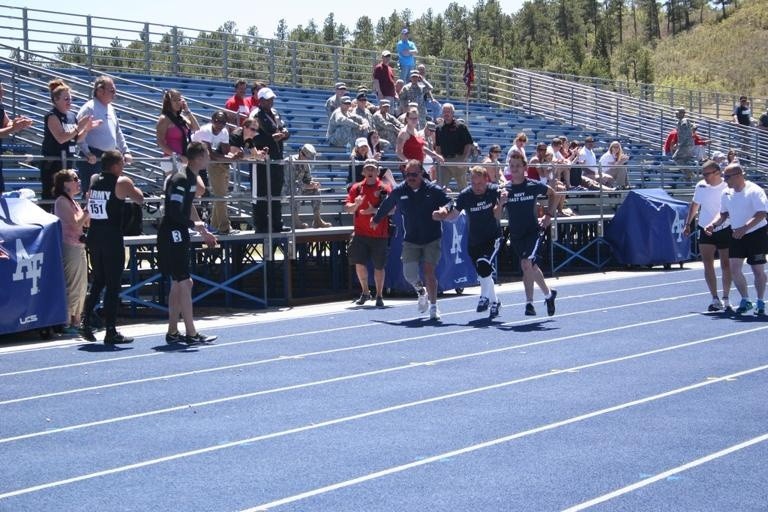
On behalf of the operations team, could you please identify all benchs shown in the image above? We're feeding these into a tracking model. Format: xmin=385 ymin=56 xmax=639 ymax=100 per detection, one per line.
xmin=426 ymin=96 xmax=767 ymax=188
xmin=0 ymin=68 xmax=398 ymax=226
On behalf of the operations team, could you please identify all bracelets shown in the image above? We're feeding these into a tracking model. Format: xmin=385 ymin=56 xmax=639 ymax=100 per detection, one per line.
xmin=73 ymin=126 xmax=81 ymax=135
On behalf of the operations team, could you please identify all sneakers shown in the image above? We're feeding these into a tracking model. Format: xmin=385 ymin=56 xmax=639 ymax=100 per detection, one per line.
xmin=477 ymin=289 xmax=557 ymax=317
xmin=166 ymin=331 xmax=186 ymax=344
xmin=78 ymin=327 xmax=96 ymax=342
xmin=708 ymin=298 xmax=765 ymax=315
xmin=104 ymin=334 xmax=134 ymax=343
xmin=356 ymin=293 xmax=371 ymax=305
xmin=186 ymin=332 xmax=217 ymax=344
xmin=430 ymin=311 xmax=440 ymax=320
xmin=376 ymin=296 xmax=383 ymax=308
xmin=416 ymin=287 xmax=428 ymax=313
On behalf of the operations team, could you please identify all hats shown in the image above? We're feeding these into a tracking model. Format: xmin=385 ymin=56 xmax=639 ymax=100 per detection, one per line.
xmin=355 ymin=137 xmax=368 ymax=147
xmin=301 ymin=143 xmax=316 ymax=160
xmin=363 ymin=158 xmax=378 ymax=169
xmin=258 ymin=87 xmax=276 ymax=100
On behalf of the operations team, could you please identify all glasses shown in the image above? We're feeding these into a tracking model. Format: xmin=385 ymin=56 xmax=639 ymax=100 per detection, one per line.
xmin=404 ymin=172 xmax=417 ymax=177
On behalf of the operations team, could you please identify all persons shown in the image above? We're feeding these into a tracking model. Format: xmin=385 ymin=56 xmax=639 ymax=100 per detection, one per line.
xmin=323 ymin=28 xmax=475 ymax=192
xmin=663 ymin=95 xmax=768 ymax=189
xmin=50 ymin=168 xmax=89 ymax=336
xmin=76 ymin=77 xmax=132 ymax=190
xmin=0 ymin=85 xmax=34 ymax=197
xmin=156 ymin=140 xmax=219 ymax=344
xmin=681 ymin=159 xmax=767 ymax=315
xmin=480 ymin=131 xmax=631 ymax=217
xmin=41 ymin=78 xmax=103 ymax=199
xmin=155 ymin=78 xmax=332 ymax=236
xmin=78 ymin=150 xmax=145 ymax=349
xmin=343 ymin=150 xmax=561 ymax=319
